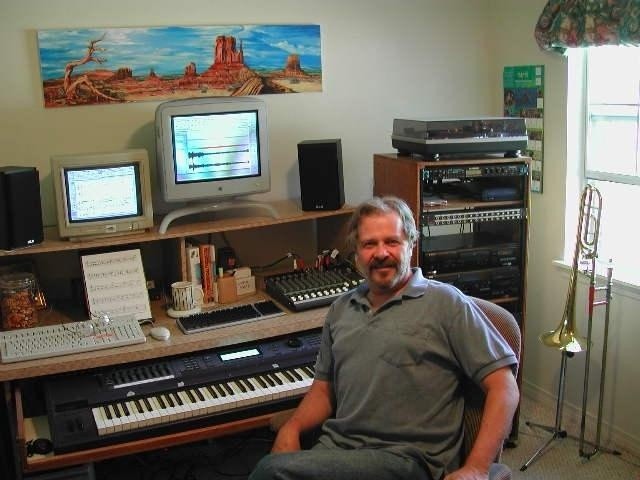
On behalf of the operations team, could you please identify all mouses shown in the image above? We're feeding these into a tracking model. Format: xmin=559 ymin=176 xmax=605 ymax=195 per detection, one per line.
xmin=150 ymin=326 xmax=172 ymax=341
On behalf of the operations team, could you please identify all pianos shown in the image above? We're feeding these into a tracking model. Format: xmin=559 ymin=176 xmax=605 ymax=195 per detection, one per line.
xmin=42 ymin=327 xmax=325 ymax=456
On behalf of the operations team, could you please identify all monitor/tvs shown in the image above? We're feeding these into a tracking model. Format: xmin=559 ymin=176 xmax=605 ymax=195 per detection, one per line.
xmin=155 ymin=97 xmax=280 ymax=235
xmin=51 ymin=147 xmax=154 ymax=245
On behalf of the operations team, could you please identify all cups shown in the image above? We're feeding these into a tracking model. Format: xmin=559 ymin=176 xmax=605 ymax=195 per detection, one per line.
xmin=171 ymin=281 xmax=204 ymax=310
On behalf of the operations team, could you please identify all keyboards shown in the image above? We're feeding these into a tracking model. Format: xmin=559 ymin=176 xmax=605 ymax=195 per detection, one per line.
xmin=176 ymin=299 xmax=287 ymax=335
xmin=1 ymin=313 xmax=147 ymax=364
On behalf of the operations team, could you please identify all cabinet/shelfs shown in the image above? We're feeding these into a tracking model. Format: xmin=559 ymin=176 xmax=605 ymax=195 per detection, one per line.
xmin=0 ymin=194 xmax=359 ymax=480
xmin=374 ymin=151 xmax=532 ymax=447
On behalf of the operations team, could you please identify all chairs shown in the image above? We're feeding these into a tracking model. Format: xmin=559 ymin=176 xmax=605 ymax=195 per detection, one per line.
xmin=456 ymin=290 xmax=522 ymax=479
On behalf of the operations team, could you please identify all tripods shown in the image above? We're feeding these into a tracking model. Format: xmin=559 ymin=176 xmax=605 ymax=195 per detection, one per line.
xmin=517 ymin=352 xmax=622 ymax=473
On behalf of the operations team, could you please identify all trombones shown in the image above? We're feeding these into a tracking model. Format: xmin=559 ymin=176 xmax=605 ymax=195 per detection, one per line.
xmin=537 ymin=184 xmax=613 ymax=457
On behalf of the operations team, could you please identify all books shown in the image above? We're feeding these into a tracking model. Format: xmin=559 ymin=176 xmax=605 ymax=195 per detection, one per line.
xmin=80 ymin=249 xmax=156 ymax=323
xmin=186 ymin=237 xmax=216 ymax=304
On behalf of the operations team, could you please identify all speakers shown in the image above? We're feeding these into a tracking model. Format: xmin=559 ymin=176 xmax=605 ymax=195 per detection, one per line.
xmin=296 ymin=139 xmax=346 ymax=213
xmin=0 ymin=165 xmax=45 ymax=251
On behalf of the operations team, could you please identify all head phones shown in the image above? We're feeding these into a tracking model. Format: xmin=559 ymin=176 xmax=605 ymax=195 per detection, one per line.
xmin=60 ymin=302 xmax=110 ymax=336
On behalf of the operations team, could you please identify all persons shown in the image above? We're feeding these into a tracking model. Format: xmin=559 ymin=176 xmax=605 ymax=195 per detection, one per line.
xmin=243 ymin=194 xmax=520 ymax=478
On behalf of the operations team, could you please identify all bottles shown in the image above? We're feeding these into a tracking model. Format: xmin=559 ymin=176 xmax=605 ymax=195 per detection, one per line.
xmin=0 ymin=272 xmax=44 ymax=330
